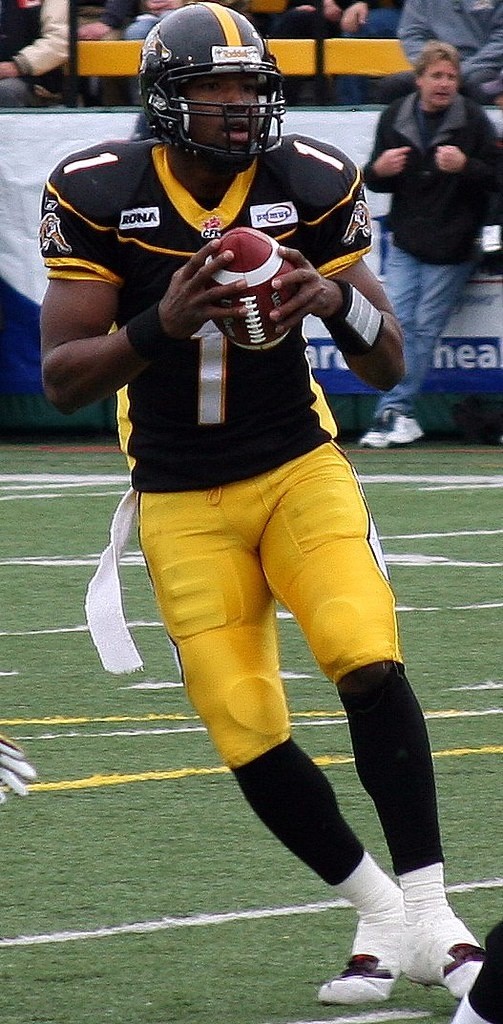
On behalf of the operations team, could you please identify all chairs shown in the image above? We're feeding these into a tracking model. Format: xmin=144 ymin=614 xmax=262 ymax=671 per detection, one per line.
xmin=321 ymin=37 xmax=414 ymax=107
xmin=60 ymin=40 xmax=146 ymax=106
xmin=263 ymin=37 xmax=320 ymax=105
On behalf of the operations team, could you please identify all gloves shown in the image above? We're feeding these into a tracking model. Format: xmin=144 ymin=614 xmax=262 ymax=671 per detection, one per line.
xmin=0 ymin=733 xmax=36 ymax=805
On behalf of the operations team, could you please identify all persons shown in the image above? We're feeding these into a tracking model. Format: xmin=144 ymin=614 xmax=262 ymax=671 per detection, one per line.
xmin=0 ymin=3 xmax=503 ymax=1024
xmin=0 ymin=0 xmax=502 ymax=447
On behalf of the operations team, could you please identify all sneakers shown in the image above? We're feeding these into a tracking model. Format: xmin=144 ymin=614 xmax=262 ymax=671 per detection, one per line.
xmin=360 ymin=427 xmax=396 ymax=448
xmin=385 ymin=412 xmax=424 ymax=444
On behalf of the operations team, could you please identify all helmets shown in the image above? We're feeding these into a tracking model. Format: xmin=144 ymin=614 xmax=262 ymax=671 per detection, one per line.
xmin=138 ymin=1 xmax=285 ymax=172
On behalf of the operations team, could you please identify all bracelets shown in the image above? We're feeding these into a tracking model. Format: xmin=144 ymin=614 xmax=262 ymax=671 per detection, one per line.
xmin=128 ymin=301 xmax=184 ymax=360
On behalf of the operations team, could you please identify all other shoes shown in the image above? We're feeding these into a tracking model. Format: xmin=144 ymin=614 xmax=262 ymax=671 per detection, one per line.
xmin=398 ymin=907 xmax=487 ymax=999
xmin=316 ymin=915 xmax=405 ymax=1005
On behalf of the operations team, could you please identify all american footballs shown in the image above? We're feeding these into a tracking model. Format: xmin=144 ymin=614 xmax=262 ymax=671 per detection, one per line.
xmin=216 ymin=228 xmax=297 ymax=350
